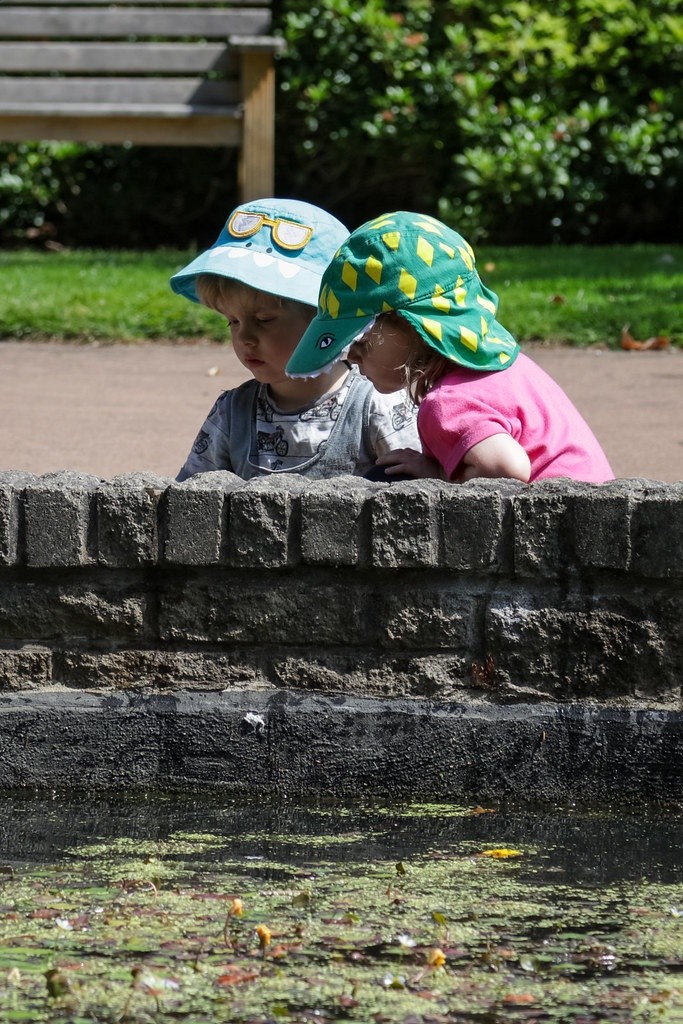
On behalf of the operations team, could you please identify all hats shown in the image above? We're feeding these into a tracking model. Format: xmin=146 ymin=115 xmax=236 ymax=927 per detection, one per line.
xmin=284 ymin=212 xmax=522 ymax=379
xmin=169 ymin=198 xmax=353 ymax=308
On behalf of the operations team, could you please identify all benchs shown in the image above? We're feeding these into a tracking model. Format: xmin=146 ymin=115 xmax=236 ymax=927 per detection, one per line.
xmin=0 ymin=0 xmax=286 ymax=205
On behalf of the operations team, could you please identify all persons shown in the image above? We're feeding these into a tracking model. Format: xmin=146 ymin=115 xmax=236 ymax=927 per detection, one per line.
xmin=285 ymin=211 xmax=616 ymax=486
xmin=170 ymin=198 xmax=422 ymax=482
